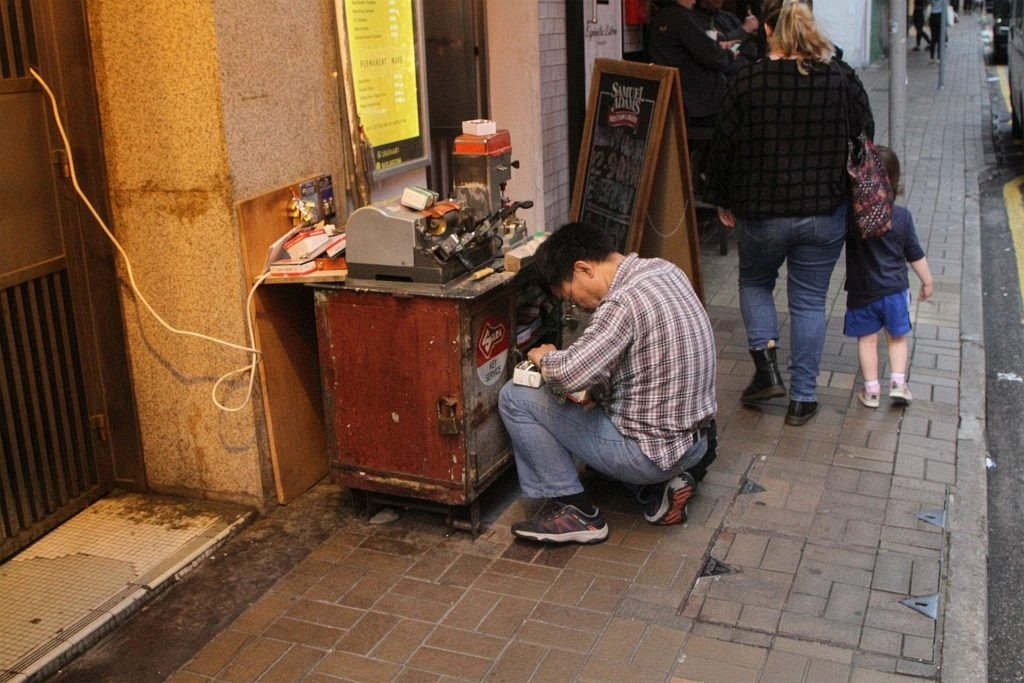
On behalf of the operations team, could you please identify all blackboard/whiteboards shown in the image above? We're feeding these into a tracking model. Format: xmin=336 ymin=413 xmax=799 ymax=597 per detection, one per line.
xmin=568 ymin=56 xmax=705 ymax=307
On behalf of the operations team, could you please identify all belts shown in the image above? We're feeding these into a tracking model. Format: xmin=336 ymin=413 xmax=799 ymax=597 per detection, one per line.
xmin=693 ymin=427 xmax=705 ymax=443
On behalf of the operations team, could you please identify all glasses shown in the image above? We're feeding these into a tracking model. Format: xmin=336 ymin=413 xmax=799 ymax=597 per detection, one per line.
xmin=564 ymin=278 xmax=576 ymax=310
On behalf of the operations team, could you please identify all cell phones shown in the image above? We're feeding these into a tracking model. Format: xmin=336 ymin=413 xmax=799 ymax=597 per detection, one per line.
xmin=731 ymin=42 xmax=741 ymax=52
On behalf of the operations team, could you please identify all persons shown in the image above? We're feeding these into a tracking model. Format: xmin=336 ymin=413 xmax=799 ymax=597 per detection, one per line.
xmin=498 ymin=222 xmax=719 ymax=543
xmin=701 ymin=0 xmax=875 ymax=426
xmin=647 ymin=0 xmax=758 ymax=127
xmin=908 ymin=0 xmax=948 ymax=63
xmin=844 ymin=146 xmax=933 ymax=408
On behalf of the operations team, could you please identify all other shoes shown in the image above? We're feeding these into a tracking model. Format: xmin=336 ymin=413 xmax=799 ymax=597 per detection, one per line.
xmin=925 ymin=46 xmax=931 ymax=50
xmin=928 ymin=58 xmax=940 ymax=64
xmin=912 ymin=47 xmax=920 ymax=52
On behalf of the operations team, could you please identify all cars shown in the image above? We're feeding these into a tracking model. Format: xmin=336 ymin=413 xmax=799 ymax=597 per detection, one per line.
xmin=984 ymin=0 xmax=1012 ymax=63
xmin=1007 ymin=0 xmax=1024 ymax=137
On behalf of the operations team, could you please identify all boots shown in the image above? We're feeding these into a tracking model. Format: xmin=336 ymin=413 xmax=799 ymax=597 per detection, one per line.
xmin=741 ymin=345 xmax=787 ymax=403
xmin=785 ymin=400 xmax=820 ymax=426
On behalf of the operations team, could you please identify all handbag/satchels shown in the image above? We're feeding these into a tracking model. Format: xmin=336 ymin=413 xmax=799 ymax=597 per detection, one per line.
xmin=835 ymin=61 xmax=893 ymax=241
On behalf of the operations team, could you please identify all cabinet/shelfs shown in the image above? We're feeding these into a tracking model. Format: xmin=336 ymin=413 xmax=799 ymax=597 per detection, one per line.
xmin=313 ymin=266 xmax=561 ymax=536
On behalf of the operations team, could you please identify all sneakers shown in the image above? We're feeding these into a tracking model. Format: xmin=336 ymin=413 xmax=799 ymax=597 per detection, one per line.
xmin=510 ymin=502 xmax=608 ymax=544
xmin=888 ymin=380 xmax=913 ymax=404
xmin=643 ymin=471 xmax=696 ymax=525
xmin=858 ymin=386 xmax=880 ymax=408
